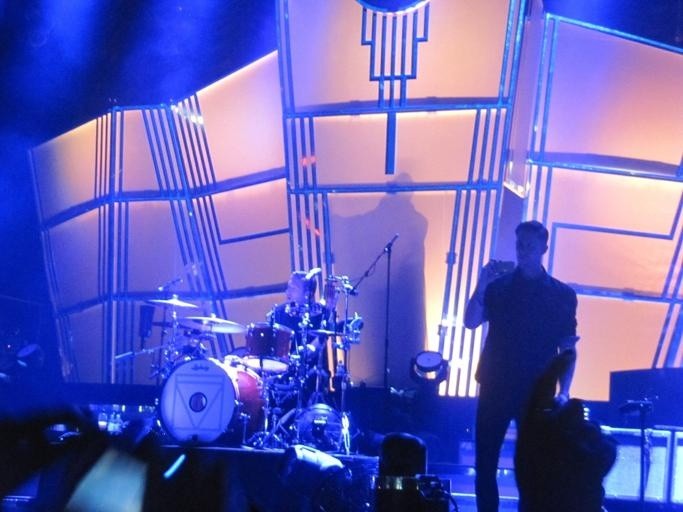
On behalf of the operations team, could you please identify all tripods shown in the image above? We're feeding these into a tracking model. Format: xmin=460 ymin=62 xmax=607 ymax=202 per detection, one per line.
xmin=244 ymin=358 xmax=344 ymax=454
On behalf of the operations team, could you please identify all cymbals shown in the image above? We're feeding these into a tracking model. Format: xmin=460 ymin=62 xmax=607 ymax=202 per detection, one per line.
xmin=149 ymin=300 xmax=198 ymax=307
xmin=177 ymin=317 xmax=248 ymax=333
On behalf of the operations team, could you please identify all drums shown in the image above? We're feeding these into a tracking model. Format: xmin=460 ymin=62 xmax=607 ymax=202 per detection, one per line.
xmin=158 ymin=358 xmax=268 ymax=445
xmin=242 ymin=322 xmax=294 ymax=372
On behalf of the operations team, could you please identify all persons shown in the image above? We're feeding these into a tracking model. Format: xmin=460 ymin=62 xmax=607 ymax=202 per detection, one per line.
xmin=512 ymin=342 xmax=620 ymax=511
xmin=265 ymin=270 xmax=332 ymax=432
xmin=0 ymin=392 xmax=233 ymax=510
xmin=460 ymin=221 xmax=580 ymax=511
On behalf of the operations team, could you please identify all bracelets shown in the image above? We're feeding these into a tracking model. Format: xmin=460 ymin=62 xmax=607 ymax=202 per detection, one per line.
xmin=527 ymin=401 xmax=554 ymax=419
xmin=556 ymin=391 xmax=570 ymax=405
xmin=473 ymin=289 xmax=485 ymax=305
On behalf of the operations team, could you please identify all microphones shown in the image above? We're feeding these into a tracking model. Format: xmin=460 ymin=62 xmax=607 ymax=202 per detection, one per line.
xmin=490 ymin=257 xmax=516 ymax=273
xmin=351 ymin=285 xmax=360 ymax=297
xmin=616 ymin=396 xmax=655 ymax=417
xmin=157 ymin=273 xmax=186 ymax=292
